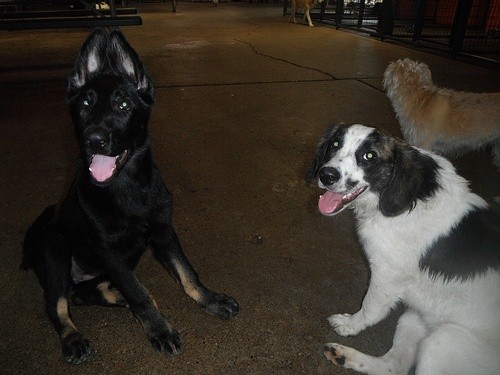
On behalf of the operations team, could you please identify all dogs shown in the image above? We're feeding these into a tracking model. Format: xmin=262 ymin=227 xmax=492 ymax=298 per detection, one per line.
xmin=381 ymin=57 xmax=500 ymax=205
xmin=288 ymin=0 xmax=322 ymax=27
xmin=20 ymin=28 xmax=240 ymax=365
xmin=307 ymin=121 xmax=500 ymax=375
